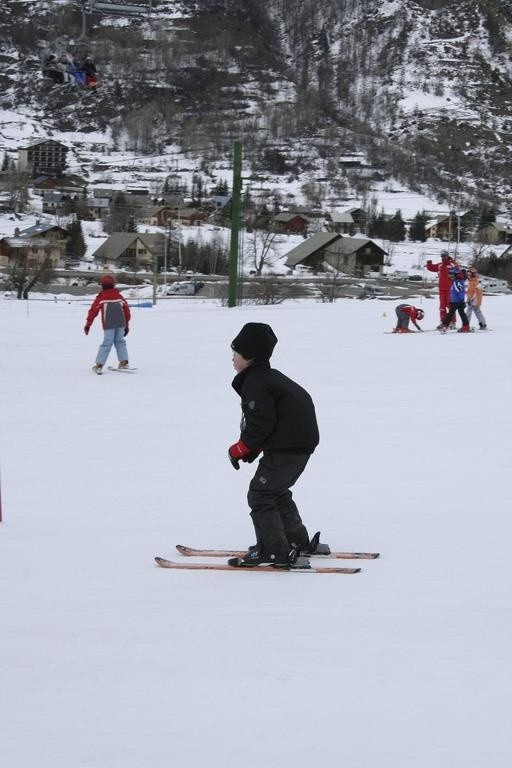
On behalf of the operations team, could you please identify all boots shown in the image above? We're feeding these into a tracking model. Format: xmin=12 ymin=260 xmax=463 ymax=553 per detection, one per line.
xmin=458 ymin=325 xmax=469 ymax=332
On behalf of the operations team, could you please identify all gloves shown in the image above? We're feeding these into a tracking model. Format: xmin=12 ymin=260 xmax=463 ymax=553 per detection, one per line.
xmin=229 ymin=438 xmax=262 ymax=470
xmin=84 ymin=325 xmax=89 ymax=333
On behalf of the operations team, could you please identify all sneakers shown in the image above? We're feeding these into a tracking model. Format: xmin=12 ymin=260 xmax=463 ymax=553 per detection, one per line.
xmin=95 ymin=363 xmax=103 ymax=370
xmin=118 ymin=360 xmax=127 ymax=368
xmin=242 ymin=526 xmax=309 ymax=566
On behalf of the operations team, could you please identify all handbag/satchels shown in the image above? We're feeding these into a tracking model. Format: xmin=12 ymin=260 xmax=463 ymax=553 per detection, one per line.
xmin=124 ymin=327 xmax=128 ymax=337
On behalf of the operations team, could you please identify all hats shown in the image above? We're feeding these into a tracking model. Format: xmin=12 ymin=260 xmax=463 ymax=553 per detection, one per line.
xmin=231 ymin=322 xmax=278 ymax=362
xmin=448 ymin=267 xmax=460 ymax=274
xmin=101 ymin=276 xmax=115 ymax=285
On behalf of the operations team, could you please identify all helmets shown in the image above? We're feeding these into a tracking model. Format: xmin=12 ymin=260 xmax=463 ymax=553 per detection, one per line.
xmin=440 ymin=251 xmax=448 ymax=256
xmin=417 ymin=309 xmax=424 ymax=320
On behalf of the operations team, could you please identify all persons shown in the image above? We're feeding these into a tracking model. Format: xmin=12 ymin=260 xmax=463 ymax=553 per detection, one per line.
xmin=84 ymin=271 xmax=132 ymax=375
xmin=226 ymin=322 xmax=321 ymax=565
xmin=57 ymin=52 xmax=76 ymax=87
xmin=73 ymin=52 xmax=100 ymax=95
xmin=394 ymin=303 xmax=425 ymax=332
xmin=436 ymin=266 xmax=470 ymax=332
xmin=42 ymin=54 xmax=64 ymax=84
xmin=427 ymin=249 xmax=461 ymax=328
xmin=466 ymin=267 xmax=487 ymax=329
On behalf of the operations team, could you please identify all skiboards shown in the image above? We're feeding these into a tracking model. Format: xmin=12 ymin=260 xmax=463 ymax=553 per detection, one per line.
xmin=154 ymin=545 xmax=379 ymax=573
xmin=385 ymin=324 xmax=494 ymax=335
xmin=93 ymin=365 xmax=138 ymax=377
